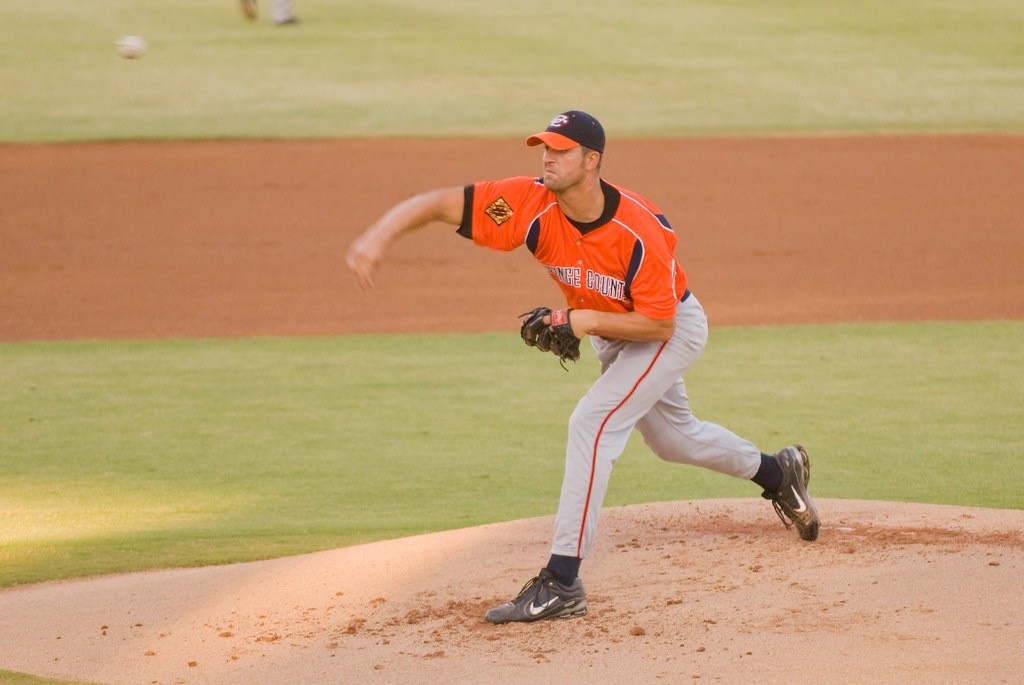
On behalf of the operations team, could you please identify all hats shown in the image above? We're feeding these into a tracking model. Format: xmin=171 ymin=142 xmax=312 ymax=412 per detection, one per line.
xmin=526 ymin=109 xmax=606 ymax=153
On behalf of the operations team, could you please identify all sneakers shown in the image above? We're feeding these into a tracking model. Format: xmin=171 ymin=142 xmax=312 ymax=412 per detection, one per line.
xmin=484 ymin=568 xmax=589 ymax=623
xmin=761 ymin=442 xmax=822 ymax=541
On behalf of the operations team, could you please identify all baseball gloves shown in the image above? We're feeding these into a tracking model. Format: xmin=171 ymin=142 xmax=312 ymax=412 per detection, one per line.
xmin=521 ymin=306 xmax=580 ymax=360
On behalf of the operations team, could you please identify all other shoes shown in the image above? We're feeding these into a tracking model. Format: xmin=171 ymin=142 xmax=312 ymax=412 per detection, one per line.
xmin=240 ymin=0 xmax=259 ymax=17
xmin=279 ymin=15 xmax=297 ymax=24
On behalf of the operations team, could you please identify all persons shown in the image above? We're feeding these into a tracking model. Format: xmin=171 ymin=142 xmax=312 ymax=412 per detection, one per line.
xmin=346 ymin=110 xmax=822 ymax=623
xmin=241 ymin=0 xmax=295 ymax=24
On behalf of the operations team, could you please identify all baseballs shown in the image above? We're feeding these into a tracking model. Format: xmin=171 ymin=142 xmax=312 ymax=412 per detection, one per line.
xmin=115 ymin=35 xmax=147 ymax=61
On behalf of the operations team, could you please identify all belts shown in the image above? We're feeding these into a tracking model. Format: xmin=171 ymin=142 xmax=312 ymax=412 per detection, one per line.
xmin=679 ymin=286 xmax=692 ymax=302
